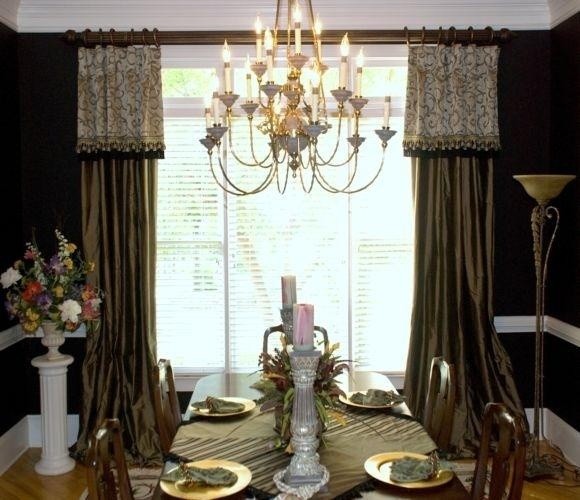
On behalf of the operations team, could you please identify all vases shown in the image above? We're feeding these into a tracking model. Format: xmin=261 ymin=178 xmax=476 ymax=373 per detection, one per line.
xmin=40 ymin=322 xmax=70 ymax=360
xmin=271 ymin=401 xmax=328 ymax=439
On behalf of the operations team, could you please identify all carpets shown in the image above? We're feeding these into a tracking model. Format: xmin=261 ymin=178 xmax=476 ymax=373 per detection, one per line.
xmin=79 ymin=462 xmax=512 ymax=500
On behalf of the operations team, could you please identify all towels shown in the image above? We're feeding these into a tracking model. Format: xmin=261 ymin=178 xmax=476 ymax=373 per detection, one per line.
xmin=186 ymin=390 xmax=243 ymax=414
xmin=386 ymin=448 xmax=460 ymax=483
xmin=349 ymin=386 xmax=407 ymax=408
xmin=162 ymin=462 xmax=239 ymax=493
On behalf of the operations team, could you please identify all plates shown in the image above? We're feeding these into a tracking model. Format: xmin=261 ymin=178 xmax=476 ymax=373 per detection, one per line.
xmin=363 ymin=449 xmax=453 ymax=488
xmin=338 ymin=389 xmax=404 ymax=410
xmin=160 ymin=459 xmax=252 ymax=500
xmin=188 ymin=396 xmax=257 ymax=418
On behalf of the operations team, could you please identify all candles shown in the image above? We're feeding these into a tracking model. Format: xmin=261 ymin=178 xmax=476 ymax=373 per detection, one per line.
xmin=281 ymin=273 xmax=301 ymax=308
xmin=291 ymin=301 xmax=317 ymax=351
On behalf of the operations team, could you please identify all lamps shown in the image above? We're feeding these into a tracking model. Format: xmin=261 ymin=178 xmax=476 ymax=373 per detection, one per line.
xmin=193 ymin=1 xmax=395 ymax=199
xmin=508 ymin=170 xmax=577 ymax=488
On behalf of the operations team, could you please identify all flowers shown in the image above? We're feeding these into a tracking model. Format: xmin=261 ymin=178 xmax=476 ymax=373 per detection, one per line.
xmin=247 ymin=330 xmax=356 ymax=454
xmin=1 ymin=225 xmax=106 ymax=335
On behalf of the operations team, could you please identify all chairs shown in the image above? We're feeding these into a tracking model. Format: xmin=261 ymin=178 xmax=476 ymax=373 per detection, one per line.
xmin=83 ymin=416 xmax=139 ymax=498
xmin=148 ymin=355 xmax=182 ymax=458
xmin=471 ymin=397 xmax=529 ymax=500
xmin=420 ymin=355 xmax=462 ymax=452
